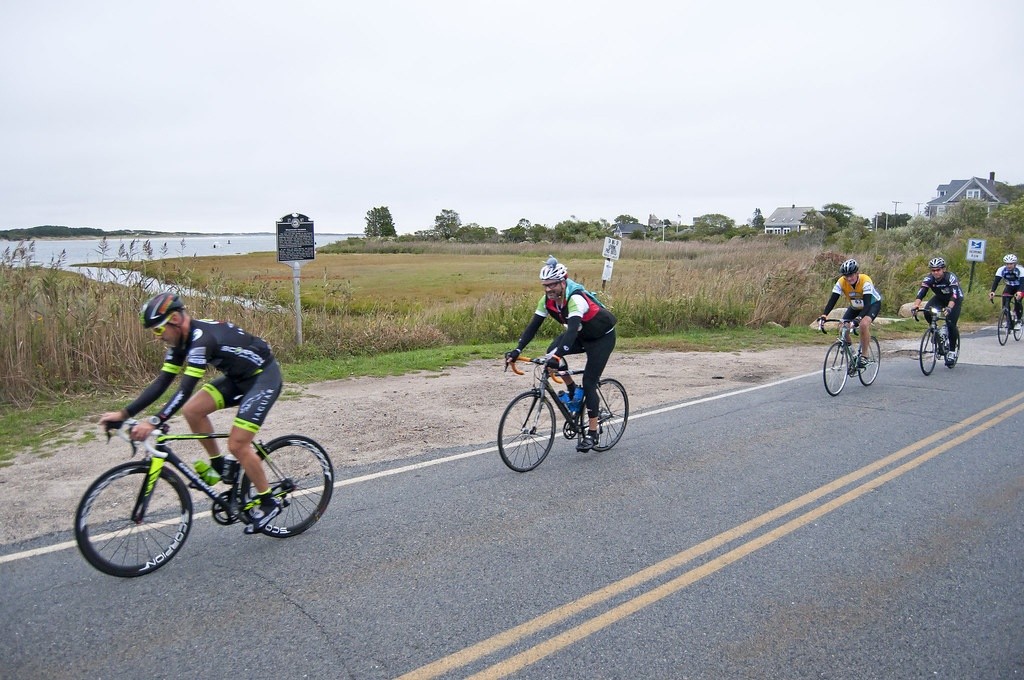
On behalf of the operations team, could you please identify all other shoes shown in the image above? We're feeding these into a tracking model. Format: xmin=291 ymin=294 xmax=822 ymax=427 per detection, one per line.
xmin=1014 ymin=321 xmax=1022 ymax=330
xmin=841 ymin=343 xmax=852 ymax=354
xmin=857 ymin=356 xmax=868 ymax=365
xmin=947 ymin=351 xmax=956 ymax=364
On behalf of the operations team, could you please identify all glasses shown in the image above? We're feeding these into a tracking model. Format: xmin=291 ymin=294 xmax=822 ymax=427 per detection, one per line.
xmin=844 ymin=273 xmax=856 ymax=278
xmin=542 ymin=279 xmax=563 ymax=289
xmin=931 ymin=268 xmax=943 ymax=274
xmin=152 ymin=311 xmax=178 ymax=335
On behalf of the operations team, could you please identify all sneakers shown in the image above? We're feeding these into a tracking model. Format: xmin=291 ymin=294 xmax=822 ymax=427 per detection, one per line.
xmin=245 ymin=497 xmax=282 ymax=534
xmin=576 ymin=434 xmax=599 ymax=449
xmin=188 ymin=467 xmax=221 ymax=488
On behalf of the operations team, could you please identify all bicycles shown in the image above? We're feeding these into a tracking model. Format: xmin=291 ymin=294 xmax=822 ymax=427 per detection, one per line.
xmin=911 ymin=307 xmax=960 ymax=376
xmin=817 ymin=317 xmax=881 ymax=396
xmin=74 ymin=421 xmax=334 ymax=578
xmin=990 ymin=294 xmax=1023 ymax=345
xmin=497 ymin=353 xmax=629 ymax=472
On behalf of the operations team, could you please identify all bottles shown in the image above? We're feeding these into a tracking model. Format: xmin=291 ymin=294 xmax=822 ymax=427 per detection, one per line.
xmin=190 ymin=457 xmax=221 ymax=486
xmin=558 ymin=390 xmax=575 ymax=412
xmin=573 ymin=385 xmax=584 ymax=412
xmin=222 ymin=452 xmax=238 ymax=484
xmin=848 ymin=343 xmax=856 ymax=355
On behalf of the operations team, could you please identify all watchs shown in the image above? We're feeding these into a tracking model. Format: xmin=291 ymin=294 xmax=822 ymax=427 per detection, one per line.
xmin=149 ymin=416 xmax=161 ymax=425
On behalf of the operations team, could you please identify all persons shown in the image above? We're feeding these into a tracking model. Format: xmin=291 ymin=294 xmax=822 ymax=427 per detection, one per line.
xmin=910 ymin=258 xmax=964 ymax=366
xmin=817 ymin=259 xmax=881 ymax=375
xmin=989 ymin=254 xmax=1024 ymax=334
xmin=505 ymin=254 xmax=618 ymax=453
xmin=97 ymin=292 xmax=282 ymax=536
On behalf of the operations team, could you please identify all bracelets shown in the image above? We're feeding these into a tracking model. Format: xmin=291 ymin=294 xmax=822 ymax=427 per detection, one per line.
xmin=819 ymin=314 xmax=827 ymax=320
xmin=552 ymin=354 xmax=560 ymax=361
xmin=855 ymin=316 xmax=862 ymax=321
xmin=516 ymin=348 xmax=521 ymax=353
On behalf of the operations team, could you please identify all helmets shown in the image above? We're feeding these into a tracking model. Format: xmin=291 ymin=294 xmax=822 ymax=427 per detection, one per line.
xmin=139 ymin=292 xmax=185 ymax=332
xmin=1003 ymin=253 xmax=1017 ymax=263
xmin=839 ymin=259 xmax=860 ymax=274
xmin=539 ymin=263 xmax=568 ymax=280
xmin=928 ymin=257 xmax=946 ymax=269
